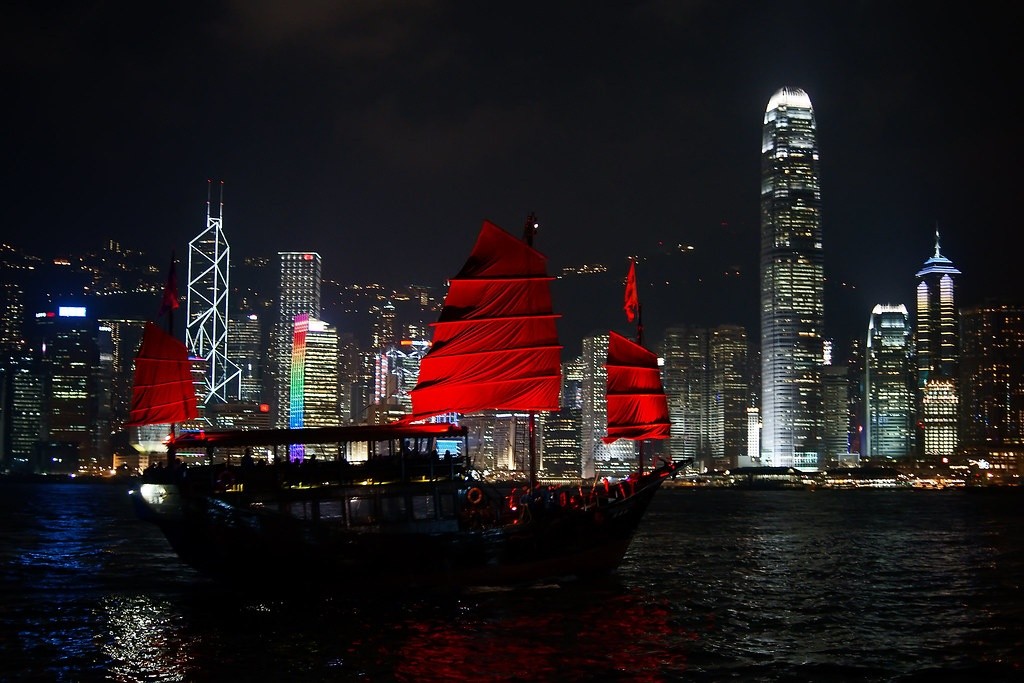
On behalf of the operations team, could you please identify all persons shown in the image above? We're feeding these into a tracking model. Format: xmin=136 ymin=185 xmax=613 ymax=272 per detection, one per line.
xmin=241 ymin=448 xmax=254 ymax=467
xmin=156 ymin=441 xmax=452 ymax=468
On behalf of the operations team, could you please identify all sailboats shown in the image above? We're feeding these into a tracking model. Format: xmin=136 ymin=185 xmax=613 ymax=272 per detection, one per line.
xmin=93 ymin=210 xmax=677 ymax=590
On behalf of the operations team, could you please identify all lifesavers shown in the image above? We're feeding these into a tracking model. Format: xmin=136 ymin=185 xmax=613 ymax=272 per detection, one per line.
xmin=468 ymin=487 xmax=483 ymax=505
